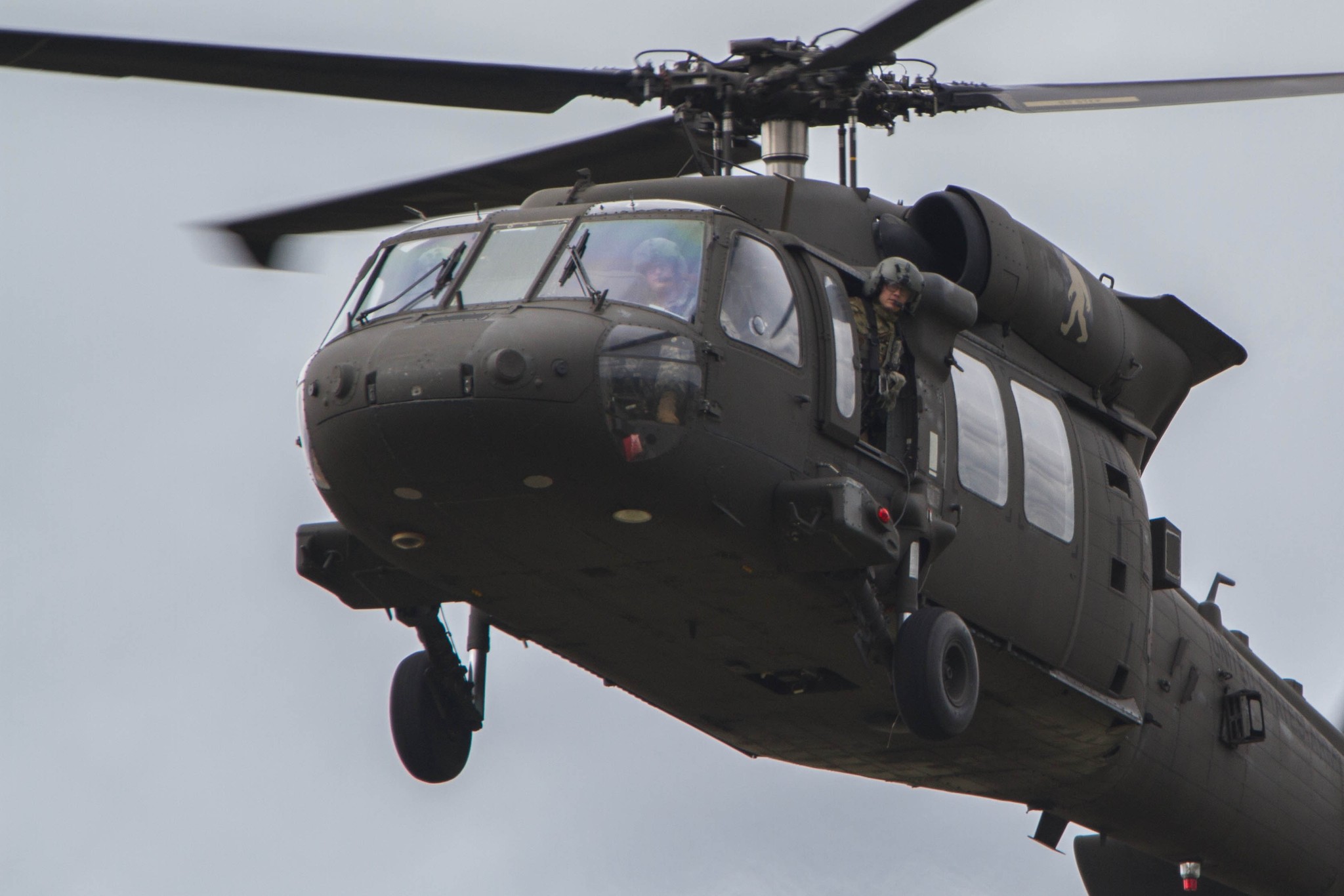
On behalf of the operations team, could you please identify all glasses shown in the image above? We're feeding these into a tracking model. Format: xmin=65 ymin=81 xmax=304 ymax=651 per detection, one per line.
xmin=886 ymin=279 xmax=899 ymax=293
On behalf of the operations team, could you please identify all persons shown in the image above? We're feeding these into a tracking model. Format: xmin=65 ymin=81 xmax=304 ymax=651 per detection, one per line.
xmin=416 ymin=246 xmax=459 ymax=310
xmin=613 ymin=238 xmax=744 ymax=425
xmin=840 ymin=256 xmax=924 ymax=441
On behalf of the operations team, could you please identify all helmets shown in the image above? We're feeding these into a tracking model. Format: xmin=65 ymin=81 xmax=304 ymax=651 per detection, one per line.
xmin=862 ymin=256 xmax=925 ymax=319
xmin=629 ymin=238 xmax=685 ymax=280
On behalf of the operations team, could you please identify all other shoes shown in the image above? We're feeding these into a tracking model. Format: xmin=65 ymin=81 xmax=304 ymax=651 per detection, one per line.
xmin=655 ymin=390 xmax=681 ymax=425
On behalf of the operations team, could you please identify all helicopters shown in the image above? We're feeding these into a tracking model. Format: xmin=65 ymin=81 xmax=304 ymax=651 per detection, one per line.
xmin=1 ymin=0 xmax=1342 ymax=896
